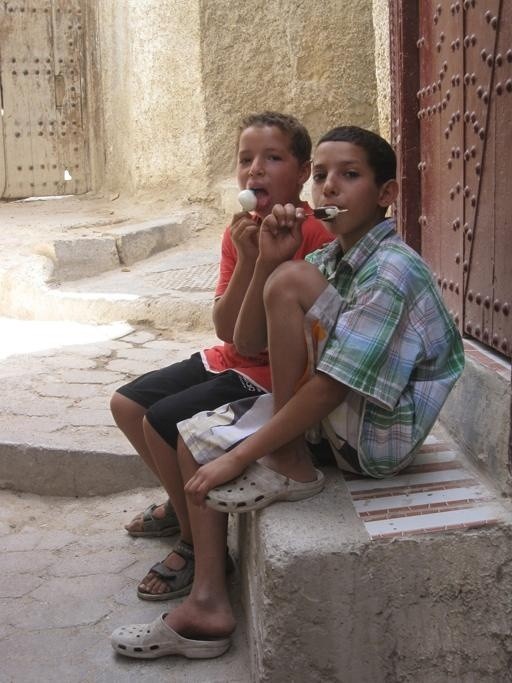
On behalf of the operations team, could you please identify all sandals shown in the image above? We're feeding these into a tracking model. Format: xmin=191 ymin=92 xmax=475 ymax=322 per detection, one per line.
xmin=136 ymin=539 xmax=236 ymax=601
xmin=126 ymin=498 xmax=180 ymax=538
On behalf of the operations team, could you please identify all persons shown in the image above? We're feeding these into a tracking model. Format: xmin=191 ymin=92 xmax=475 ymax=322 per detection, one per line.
xmin=110 ymin=124 xmax=467 ymax=658
xmin=110 ymin=109 xmax=338 ymax=602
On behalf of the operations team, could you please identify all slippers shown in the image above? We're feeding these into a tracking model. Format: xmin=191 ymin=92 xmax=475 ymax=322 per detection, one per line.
xmin=204 ymin=458 xmax=327 ymax=515
xmin=110 ymin=612 xmax=233 ymax=660
